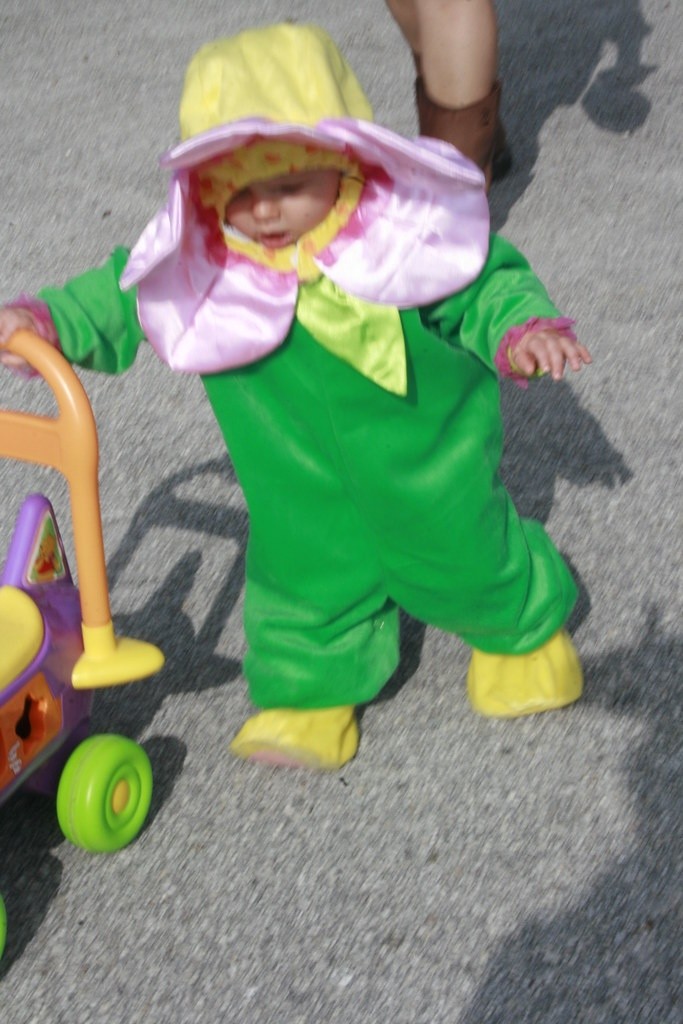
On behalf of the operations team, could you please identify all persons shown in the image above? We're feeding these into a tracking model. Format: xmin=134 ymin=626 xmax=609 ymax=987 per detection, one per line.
xmin=0 ymin=22 xmax=595 ymax=770
xmin=385 ymin=0 xmax=513 ymax=194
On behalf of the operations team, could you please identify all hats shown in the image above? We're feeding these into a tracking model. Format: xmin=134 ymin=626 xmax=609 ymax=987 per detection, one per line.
xmin=117 ymin=22 xmax=491 ymax=401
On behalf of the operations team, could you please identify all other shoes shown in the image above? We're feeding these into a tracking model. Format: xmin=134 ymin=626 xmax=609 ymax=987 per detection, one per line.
xmin=400 ymin=42 xmax=510 ymax=186
